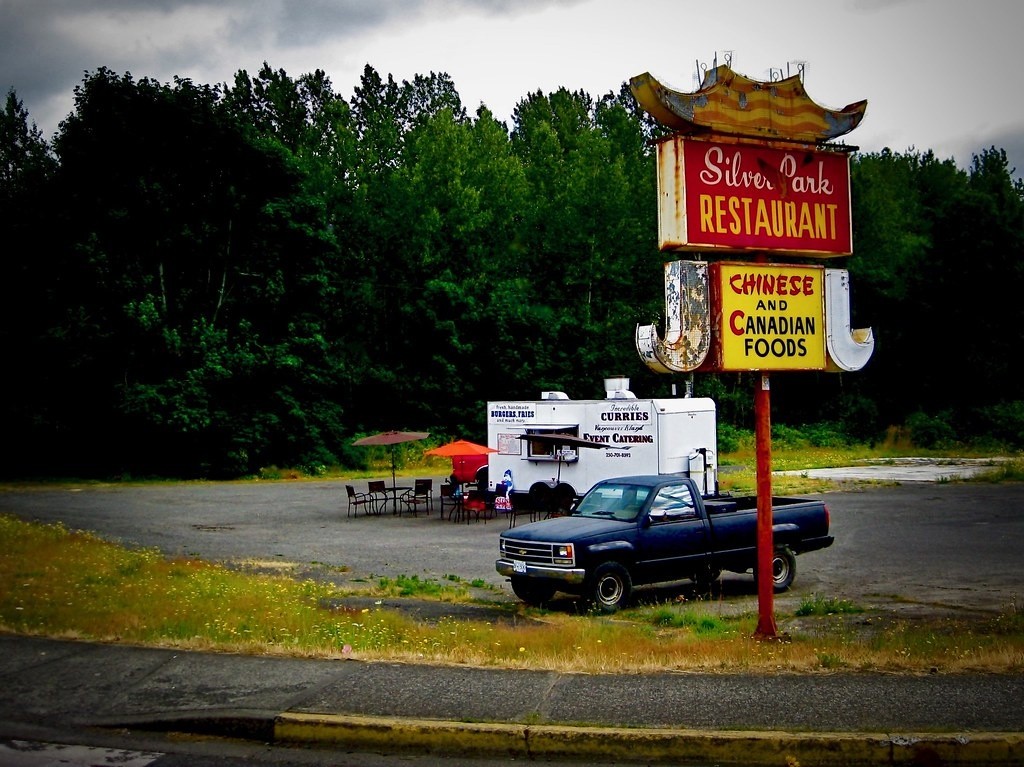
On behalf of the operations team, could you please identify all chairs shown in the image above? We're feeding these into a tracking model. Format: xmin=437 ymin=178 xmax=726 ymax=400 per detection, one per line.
xmin=368 ymin=480 xmax=389 ymax=514
xmin=399 ymin=477 xmax=434 ymax=518
xmin=509 ymin=491 xmax=536 ymax=529
xmin=456 ymin=489 xmax=488 ymax=525
xmin=344 ymin=484 xmax=370 ymax=515
xmin=483 ymin=483 xmax=507 ymax=517
xmin=440 ymin=483 xmax=463 ymax=520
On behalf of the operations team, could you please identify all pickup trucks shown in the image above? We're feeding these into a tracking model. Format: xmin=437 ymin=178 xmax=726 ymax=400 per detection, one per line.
xmin=493 ymin=474 xmax=836 ymax=618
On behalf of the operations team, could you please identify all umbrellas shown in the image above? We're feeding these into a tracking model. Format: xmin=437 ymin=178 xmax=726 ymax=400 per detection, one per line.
xmin=424 ymin=439 xmax=500 ymax=521
xmin=351 ymin=430 xmax=430 ymax=515
xmin=515 ymin=433 xmax=611 ymax=482
